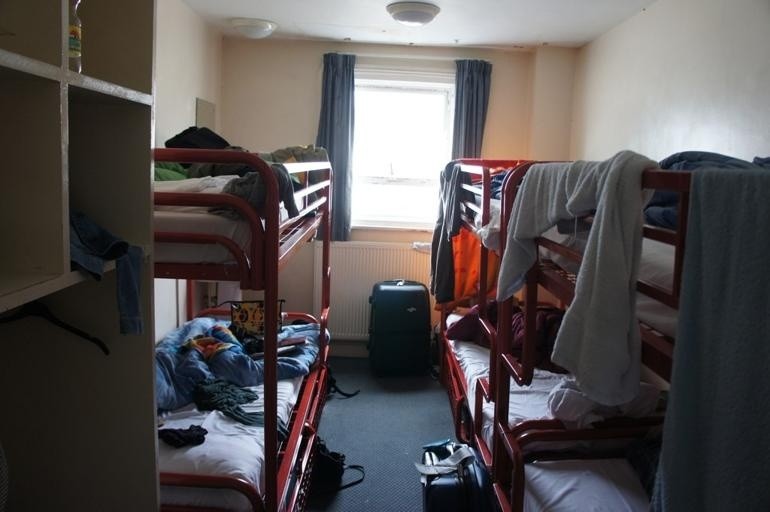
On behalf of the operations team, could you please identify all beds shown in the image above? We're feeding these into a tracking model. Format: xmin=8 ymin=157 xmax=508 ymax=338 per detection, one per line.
xmin=434 ymin=158 xmax=770 ymax=512
xmin=153 ymin=148 xmax=333 ymax=512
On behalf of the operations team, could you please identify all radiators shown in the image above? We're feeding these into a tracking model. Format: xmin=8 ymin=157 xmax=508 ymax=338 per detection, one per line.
xmin=312 ymin=241 xmax=431 ymax=341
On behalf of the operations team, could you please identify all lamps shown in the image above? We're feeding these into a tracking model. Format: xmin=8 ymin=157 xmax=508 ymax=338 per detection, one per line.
xmin=385 ymin=2 xmax=440 ymax=27
xmin=231 ymin=19 xmax=278 ymax=39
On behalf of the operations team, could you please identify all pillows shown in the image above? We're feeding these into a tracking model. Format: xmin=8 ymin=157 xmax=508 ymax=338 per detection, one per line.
xmin=546 ymin=378 xmax=659 ymax=429
xmin=154 ymin=173 xmax=238 ymax=211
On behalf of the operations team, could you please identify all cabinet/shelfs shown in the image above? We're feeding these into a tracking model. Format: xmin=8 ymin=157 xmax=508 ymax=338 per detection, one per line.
xmin=0 ymin=0 xmax=160 ymax=512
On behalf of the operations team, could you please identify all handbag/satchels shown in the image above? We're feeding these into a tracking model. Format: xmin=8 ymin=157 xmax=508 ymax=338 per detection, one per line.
xmin=307 ymin=444 xmax=345 ymax=511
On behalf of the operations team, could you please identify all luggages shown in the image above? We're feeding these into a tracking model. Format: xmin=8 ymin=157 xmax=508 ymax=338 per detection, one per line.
xmin=420 ymin=440 xmax=492 ymax=511
xmin=368 ymin=280 xmax=433 ymax=377
